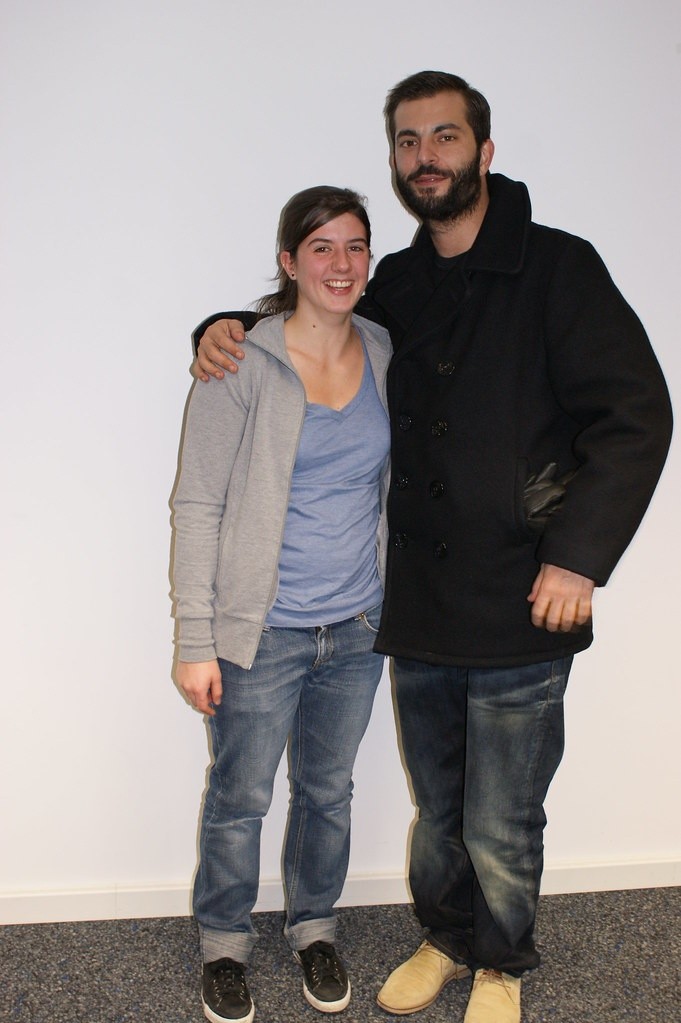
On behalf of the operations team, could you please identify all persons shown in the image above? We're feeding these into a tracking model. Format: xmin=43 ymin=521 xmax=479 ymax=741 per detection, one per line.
xmin=189 ymin=69 xmax=675 ymax=1022
xmin=166 ymin=185 xmax=395 ymax=1023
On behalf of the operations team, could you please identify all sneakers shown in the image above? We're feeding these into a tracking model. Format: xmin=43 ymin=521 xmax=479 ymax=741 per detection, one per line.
xmin=292 ymin=941 xmax=351 ymax=1012
xmin=201 ymin=958 xmax=255 ymax=1023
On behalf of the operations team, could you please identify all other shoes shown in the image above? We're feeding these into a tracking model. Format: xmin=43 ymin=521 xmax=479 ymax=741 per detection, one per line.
xmin=463 ymin=966 xmax=522 ymax=1023
xmin=377 ymin=937 xmax=473 ymax=1014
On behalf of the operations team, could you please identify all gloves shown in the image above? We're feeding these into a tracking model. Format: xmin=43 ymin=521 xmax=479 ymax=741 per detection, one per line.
xmin=525 ymin=462 xmax=569 ymax=529
